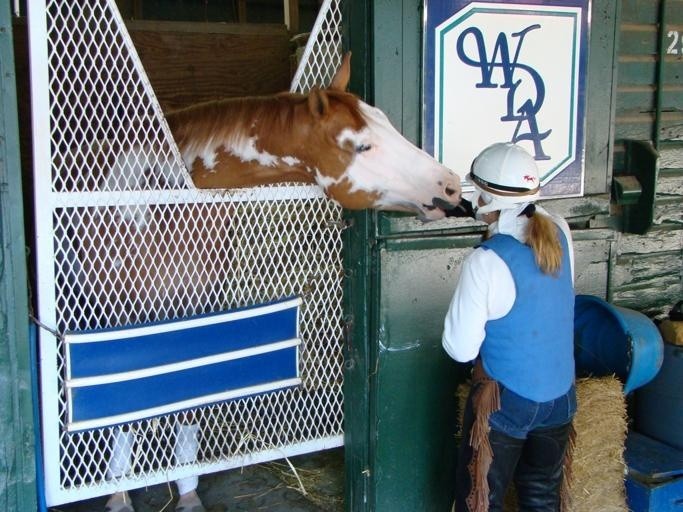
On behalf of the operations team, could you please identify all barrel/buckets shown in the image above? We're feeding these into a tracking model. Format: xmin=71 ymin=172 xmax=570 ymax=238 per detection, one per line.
xmin=574 ymin=293 xmax=664 ymax=398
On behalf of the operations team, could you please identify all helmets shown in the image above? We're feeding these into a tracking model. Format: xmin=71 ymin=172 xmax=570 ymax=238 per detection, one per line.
xmin=465 ymin=142 xmax=540 ymax=203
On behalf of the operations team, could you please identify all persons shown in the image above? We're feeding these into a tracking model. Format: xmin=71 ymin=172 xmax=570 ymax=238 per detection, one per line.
xmin=439 ymin=141 xmax=577 ymax=511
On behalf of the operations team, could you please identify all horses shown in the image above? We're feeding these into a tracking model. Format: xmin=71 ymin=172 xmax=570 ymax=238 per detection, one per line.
xmin=51 ymin=51 xmax=464 ymax=512
